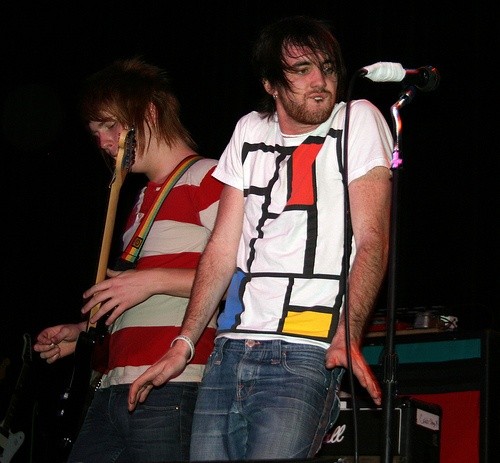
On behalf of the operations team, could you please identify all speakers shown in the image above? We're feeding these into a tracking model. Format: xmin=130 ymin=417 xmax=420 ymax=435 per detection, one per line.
xmin=312 ymin=396 xmax=443 ymax=463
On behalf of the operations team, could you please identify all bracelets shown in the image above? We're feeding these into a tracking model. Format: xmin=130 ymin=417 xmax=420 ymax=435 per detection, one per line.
xmin=170 ymin=335 xmax=195 ymax=363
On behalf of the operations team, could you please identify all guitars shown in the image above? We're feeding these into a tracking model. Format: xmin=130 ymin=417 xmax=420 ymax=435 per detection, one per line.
xmin=33 ymin=128 xmax=138 ymax=462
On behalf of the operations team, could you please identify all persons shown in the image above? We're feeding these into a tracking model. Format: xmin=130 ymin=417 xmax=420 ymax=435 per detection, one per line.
xmin=128 ymin=17 xmax=394 ymax=463
xmin=34 ymin=58 xmax=224 ymax=463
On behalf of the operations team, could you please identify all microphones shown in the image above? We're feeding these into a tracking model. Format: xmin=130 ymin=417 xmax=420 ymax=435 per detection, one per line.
xmin=358 ymin=61 xmax=440 ymax=93
